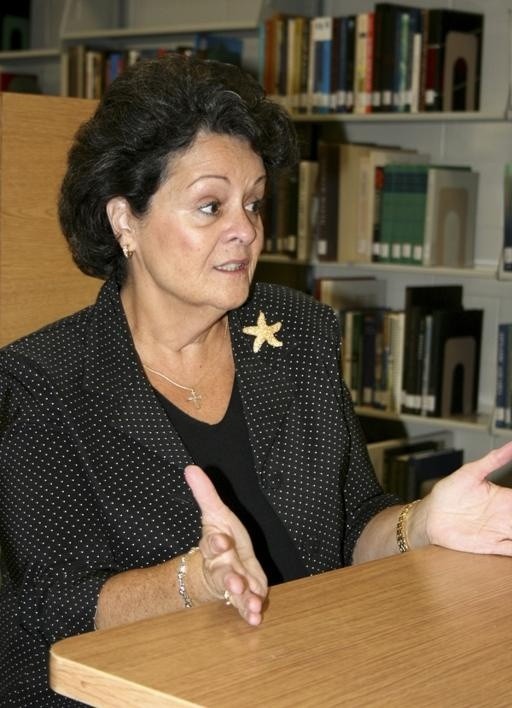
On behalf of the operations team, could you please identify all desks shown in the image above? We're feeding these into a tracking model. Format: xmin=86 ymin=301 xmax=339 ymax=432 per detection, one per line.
xmin=45 ymin=545 xmax=511 ymax=705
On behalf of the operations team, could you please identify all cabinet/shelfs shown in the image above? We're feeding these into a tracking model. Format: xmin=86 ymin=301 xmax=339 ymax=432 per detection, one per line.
xmin=0 ymin=3 xmax=510 ymax=438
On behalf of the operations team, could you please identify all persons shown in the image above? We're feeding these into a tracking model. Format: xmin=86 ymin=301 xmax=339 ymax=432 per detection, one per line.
xmin=0 ymin=55 xmax=512 ymax=708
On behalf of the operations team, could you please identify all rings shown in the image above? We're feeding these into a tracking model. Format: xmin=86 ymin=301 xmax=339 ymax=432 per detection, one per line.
xmin=222 ymin=588 xmax=232 ymax=607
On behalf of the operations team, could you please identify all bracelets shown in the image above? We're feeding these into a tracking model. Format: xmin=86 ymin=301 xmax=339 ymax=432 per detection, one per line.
xmin=177 ymin=546 xmax=201 ymax=611
xmin=395 ymin=500 xmax=421 ymax=552
